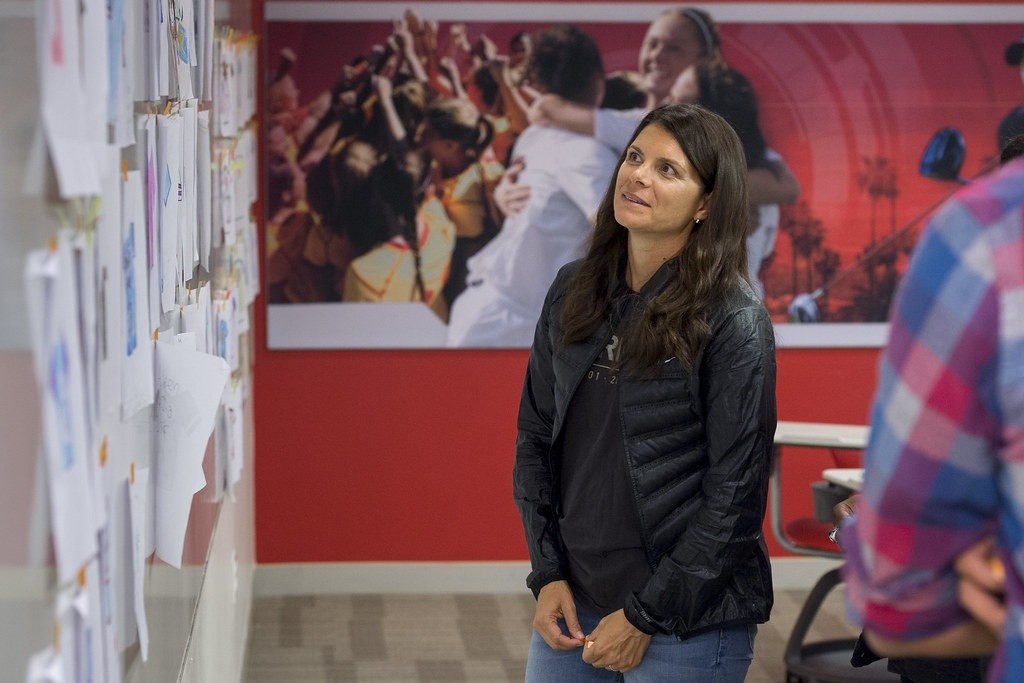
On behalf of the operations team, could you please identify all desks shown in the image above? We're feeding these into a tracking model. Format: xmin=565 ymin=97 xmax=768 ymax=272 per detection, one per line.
xmin=769 ymin=421 xmax=872 ymax=559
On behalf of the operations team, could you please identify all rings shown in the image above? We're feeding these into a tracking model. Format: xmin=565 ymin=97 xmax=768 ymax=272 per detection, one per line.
xmin=609 ymin=665 xmax=618 ymax=672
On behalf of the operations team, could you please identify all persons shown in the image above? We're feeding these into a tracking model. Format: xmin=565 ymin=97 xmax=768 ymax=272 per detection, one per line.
xmin=834 ymin=100 xmax=1024 ymax=682
xmin=511 ymin=104 xmax=774 ymax=683
xmin=267 ymin=5 xmax=803 ymax=335
